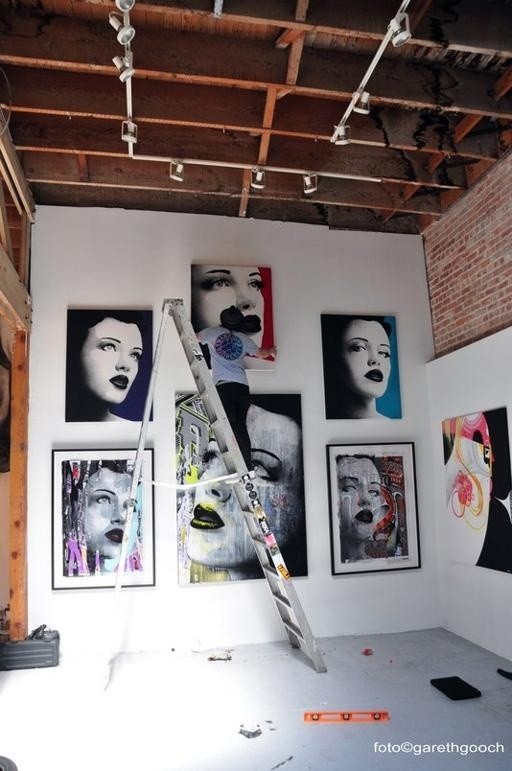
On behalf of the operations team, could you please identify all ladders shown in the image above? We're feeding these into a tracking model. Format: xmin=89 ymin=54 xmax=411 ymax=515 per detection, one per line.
xmin=99 ymin=298 xmax=328 ymax=692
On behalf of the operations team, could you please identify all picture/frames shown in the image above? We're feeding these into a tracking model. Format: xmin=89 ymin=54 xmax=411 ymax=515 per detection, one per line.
xmin=326 ymin=442 xmax=421 ymax=576
xmin=51 ymin=448 xmax=157 ymax=592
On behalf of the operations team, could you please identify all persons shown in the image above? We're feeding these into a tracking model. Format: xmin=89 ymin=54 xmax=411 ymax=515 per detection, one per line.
xmin=66 ymin=310 xmax=145 ymax=419
xmin=74 ymin=459 xmax=140 ymax=575
xmin=336 ymin=456 xmax=386 ymax=560
xmin=192 ymin=264 xmax=266 ymax=354
xmin=322 ymin=314 xmax=395 ymax=420
xmin=184 ymin=395 xmax=303 ymax=578
xmin=195 ymin=308 xmax=278 ymax=481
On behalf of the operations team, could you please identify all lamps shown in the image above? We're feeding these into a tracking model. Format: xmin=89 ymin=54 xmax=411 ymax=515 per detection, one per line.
xmin=330 ymin=0 xmax=415 ymax=146
xmin=107 ymin=0 xmax=139 ymax=161
xmin=132 ymin=153 xmax=381 ymax=197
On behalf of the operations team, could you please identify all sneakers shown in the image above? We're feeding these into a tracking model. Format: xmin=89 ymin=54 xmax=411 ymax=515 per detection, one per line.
xmin=224 ymin=469 xmax=256 ymax=484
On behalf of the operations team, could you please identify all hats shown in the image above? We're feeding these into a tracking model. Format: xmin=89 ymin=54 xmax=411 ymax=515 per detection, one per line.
xmin=220 ymin=305 xmax=246 ymax=329
